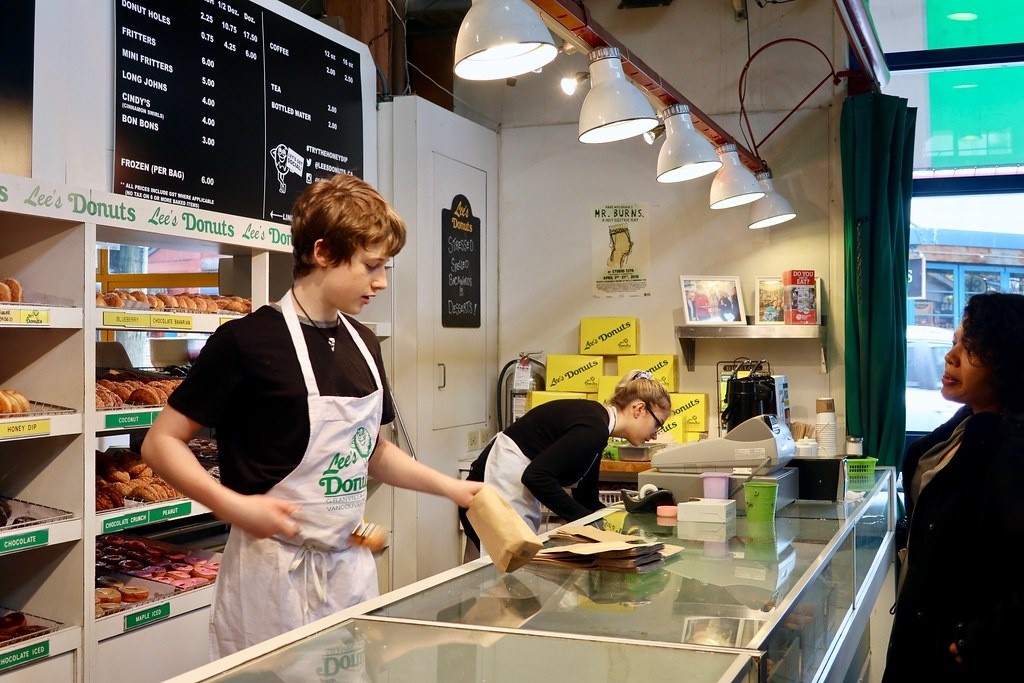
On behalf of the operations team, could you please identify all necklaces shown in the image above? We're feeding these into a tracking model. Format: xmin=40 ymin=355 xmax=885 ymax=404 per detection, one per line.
xmin=291 ymin=285 xmax=338 ymax=352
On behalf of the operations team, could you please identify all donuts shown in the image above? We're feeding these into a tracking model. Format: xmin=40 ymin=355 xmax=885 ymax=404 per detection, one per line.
xmin=188 ymin=439 xmax=221 ymax=482
xmin=0 ymin=613 xmax=51 ymax=647
xmin=0 ymin=498 xmax=38 ymax=529
xmin=95 ymin=534 xmax=220 ymax=619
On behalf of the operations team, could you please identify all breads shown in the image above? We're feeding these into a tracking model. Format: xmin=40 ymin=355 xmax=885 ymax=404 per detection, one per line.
xmin=0 ymin=389 xmax=30 ymax=418
xmin=95 ymin=439 xmax=185 ymax=511
xmin=0 ymin=278 xmax=24 ymax=302
xmin=96 ymin=371 xmax=182 ymax=411
xmin=96 ymin=291 xmax=251 ymax=314
xmin=766 ymin=599 xmax=815 ymax=630
xmin=350 ymin=524 xmax=388 ymax=552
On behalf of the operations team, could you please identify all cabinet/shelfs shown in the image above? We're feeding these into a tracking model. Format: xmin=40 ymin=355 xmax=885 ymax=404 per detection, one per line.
xmin=0 ymin=174 xmax=296 ymax=683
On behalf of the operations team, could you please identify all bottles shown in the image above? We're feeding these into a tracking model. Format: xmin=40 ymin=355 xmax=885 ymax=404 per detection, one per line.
xmin=794 ymin=435 xmax=819 ymax=457
xmin=846 ymin=436 xmax=864 ymax=456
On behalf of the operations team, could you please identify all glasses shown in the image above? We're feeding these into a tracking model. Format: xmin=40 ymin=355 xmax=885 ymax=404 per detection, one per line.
xmin=633 ymin=404 xmax=664 ymax=434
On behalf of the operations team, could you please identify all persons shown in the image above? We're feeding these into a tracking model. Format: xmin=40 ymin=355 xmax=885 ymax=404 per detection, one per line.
xmin=760 ymin=297 xmax=784 ymax=321
xmin=457 ymin=370 xmax=671 ymax=559
xmin=141 ymin=173 xmax=486 ymax=663
xmin=685 ymin=285 xmax=741 ymax=321
xmin=880 ymin=293 xmax=1024 ymax=683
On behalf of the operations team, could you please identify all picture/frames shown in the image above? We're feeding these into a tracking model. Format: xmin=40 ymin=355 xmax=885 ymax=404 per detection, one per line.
xmin=755 ymin=276 xmax=821 ymax=325
xmin=680 ymin=275 xmax=748 ymax=325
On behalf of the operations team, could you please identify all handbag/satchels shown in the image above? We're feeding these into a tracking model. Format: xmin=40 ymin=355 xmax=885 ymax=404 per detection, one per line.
xmin=721 ymin=360 xmax=778 ymax=433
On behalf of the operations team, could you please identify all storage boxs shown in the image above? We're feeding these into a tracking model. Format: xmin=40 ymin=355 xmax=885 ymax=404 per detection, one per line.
xmin=782 ymin=270 xmax=816 ymax=324
xmin=526 ymin=318 xmax=710 ymax=446
xmin=847 ymin=456 xmax=879 ymax=476
xmin=678 ymin=497 xmax=737 ymax=524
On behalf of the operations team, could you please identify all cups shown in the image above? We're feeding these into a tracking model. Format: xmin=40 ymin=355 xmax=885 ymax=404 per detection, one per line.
xmin=815 ymin=398 xmax=837 ymax=458
xmin=743 ymin=482 xmax=779 ymax=523
xmin=746 ymin=523 xmax=778 ymax=564
xmin=698 ymin=471 xmax=732 ymax=499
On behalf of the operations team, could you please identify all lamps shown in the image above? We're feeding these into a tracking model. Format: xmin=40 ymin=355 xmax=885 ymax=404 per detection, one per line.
xmin=579 ymin=44 xmax=659 ymax=144
xmin=656 ymin=103 xmax=723 ymax=183
xmin=454 ymin=0 xmax=558 ymax=81
xmin=749 ymin=172 xmax=797 ymax=229
xmin=532 ymin=38 xmax=578 ymax=74
xmin=709 ymin=143 xmax=766 ymax=209
xmin=560 ymin=72 xmax=590 ymax=96
xmin=644 ymin=124 xmax=665 ymax=146
